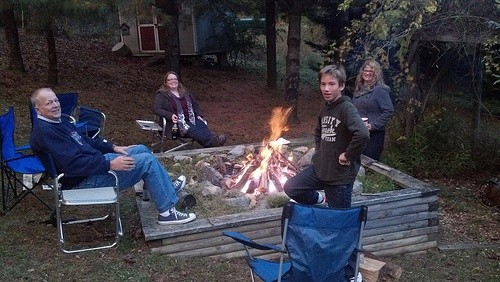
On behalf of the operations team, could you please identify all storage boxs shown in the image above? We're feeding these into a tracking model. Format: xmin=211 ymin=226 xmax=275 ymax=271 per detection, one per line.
xmin=360 ymin=256 xmax=387 ymax=282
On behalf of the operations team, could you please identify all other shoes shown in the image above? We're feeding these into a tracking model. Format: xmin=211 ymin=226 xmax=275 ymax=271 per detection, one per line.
xmin=313 ymin=191 xmax=326 ymax=209
xmin=204 ymin=134 xmax=227 ymax=148
xmin=349 ymin=272 xmax=363 ymax=282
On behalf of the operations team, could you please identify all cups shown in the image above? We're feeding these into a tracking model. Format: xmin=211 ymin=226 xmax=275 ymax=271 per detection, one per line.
xmin=361 ymin=117 xmax=368 ymax=126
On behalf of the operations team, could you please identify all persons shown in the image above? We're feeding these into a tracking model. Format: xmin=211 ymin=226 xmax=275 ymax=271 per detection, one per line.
xmin=29 ymin=88 xmax=196 ymax=225
xmin=352 ymin=60 xmax=394 ymax=161
xmin=151 ymin=70 xmax=227 ymax=148
xmin=283 ymin=65 xmax=371 ymax=282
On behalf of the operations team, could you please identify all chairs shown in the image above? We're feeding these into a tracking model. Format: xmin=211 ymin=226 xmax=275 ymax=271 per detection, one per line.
xmin=221 ymin=201 xmax=368 ymax=282
xmin=0 ymin=92 xmax=123 ymax=253
xmin=135 ymin=114 xmax=207 ymax=154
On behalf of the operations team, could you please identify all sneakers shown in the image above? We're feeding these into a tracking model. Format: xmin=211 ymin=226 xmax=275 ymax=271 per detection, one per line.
xmin=157 ymin=207 xmax=196 ymax=226
xmin=174 ymin=175 xmax=186 ymax=194
